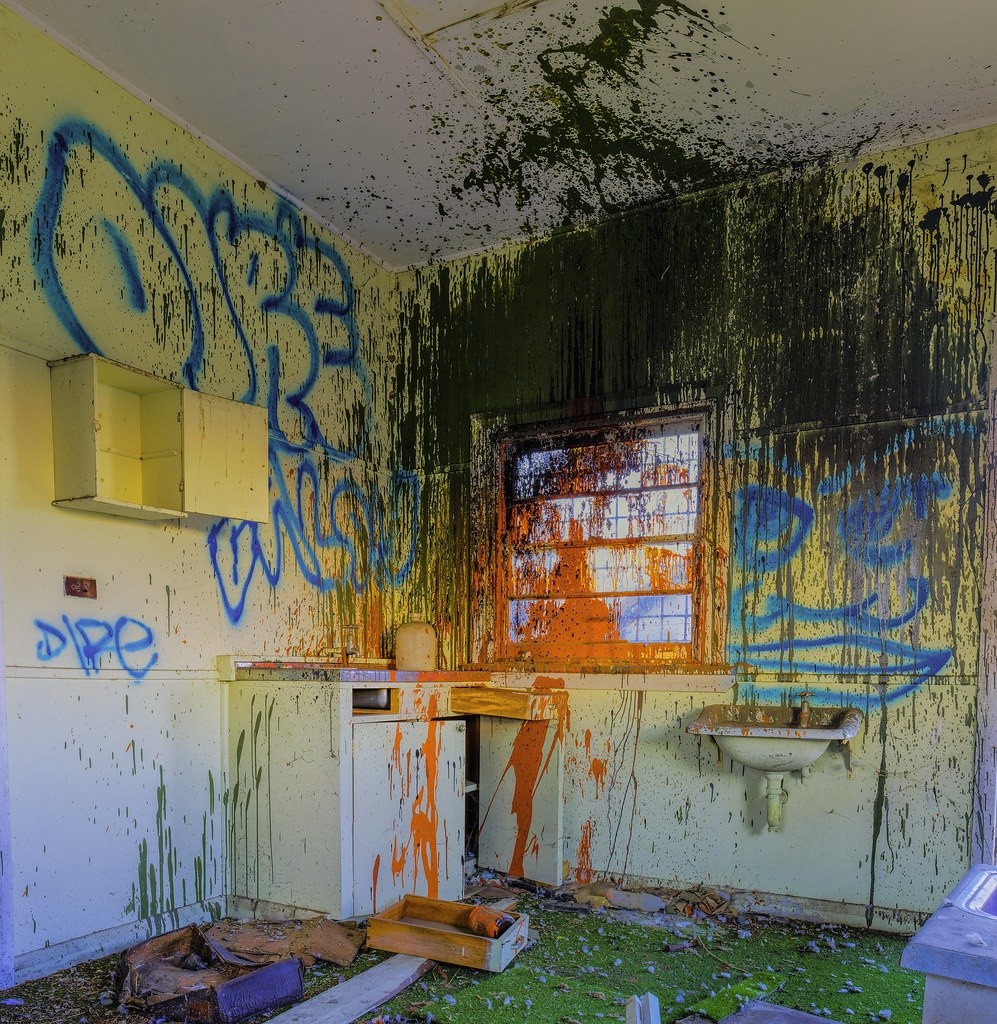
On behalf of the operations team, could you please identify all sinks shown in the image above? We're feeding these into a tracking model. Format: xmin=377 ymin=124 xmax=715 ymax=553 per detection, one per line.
xmin=685 ymin=704 xmax=866 ymax=771
xmin=948 ymin=870 xmax=996 ymax=920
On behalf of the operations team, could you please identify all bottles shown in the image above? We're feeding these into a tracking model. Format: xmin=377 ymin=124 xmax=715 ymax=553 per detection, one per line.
xmin=607 ymin=891 xmax=664 ymax=911
xmin=468 ymin=905 xmax=515 ymax=938
xmin=395 ymin=614 xmax=436 ymax=672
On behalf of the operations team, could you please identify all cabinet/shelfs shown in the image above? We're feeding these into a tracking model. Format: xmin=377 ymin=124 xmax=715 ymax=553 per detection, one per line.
xmin=210 ymin=669 xmax=570 ymax=918
xmin=43 ymin=348 xmax=270 ymax=529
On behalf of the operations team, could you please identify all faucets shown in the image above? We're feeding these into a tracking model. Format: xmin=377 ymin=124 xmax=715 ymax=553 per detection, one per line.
xmin=340 ymin=624 xmax=360 ymax=659
xmin=796 ymin=692 xmax=815 ymax=726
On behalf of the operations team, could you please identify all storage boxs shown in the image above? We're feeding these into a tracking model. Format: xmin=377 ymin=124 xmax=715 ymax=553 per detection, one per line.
xmin=360 ymin=893 xmax=540 ymax=973
